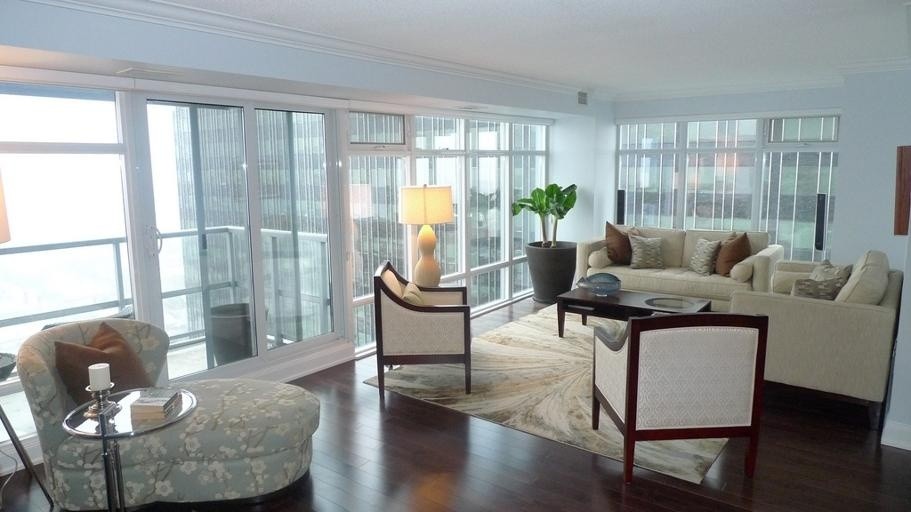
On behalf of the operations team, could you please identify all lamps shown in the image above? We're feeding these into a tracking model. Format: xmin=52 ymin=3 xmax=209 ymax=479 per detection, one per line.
xmin=397 ymin=183 xmax=454 ymax=289
xmin=321 ymin=182 xmax=374 ymax=220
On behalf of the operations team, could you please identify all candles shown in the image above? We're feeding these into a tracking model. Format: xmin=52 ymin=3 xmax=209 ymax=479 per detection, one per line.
xmin=87 ymin=362 xmax=111 ymax=391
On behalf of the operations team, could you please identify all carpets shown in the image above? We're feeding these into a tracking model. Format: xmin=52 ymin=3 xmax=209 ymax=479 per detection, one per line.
xmin=362 ymin=300 xmax=736 ymax=485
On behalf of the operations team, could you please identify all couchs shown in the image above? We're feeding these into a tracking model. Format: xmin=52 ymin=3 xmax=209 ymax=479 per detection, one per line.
xmin=578 ymin=223 xmax=785 ymax=312
xmin=17 ymin=317 xmax=322 ymax=510
xmin=729 ymin=249 xmax=905 ymax=419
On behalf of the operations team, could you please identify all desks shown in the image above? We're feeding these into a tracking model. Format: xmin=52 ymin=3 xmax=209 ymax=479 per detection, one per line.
xmin=62 ymin=386 xmax=197 ymax=510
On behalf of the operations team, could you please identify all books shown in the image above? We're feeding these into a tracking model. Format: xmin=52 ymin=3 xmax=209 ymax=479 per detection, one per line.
xmin=131 ymin=386 xmax=183 ymax=419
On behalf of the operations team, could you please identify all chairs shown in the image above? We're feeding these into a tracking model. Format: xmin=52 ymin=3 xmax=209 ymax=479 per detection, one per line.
xmin=373 ymin=259 xmax=472 ymax=398
xmin=592 ymin=314 xmax=771 ymax=484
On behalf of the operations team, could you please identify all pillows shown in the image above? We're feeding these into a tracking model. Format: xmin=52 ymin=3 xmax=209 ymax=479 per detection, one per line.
xmin=792 ymin=278 xmax=846 ymax=303
xmin=716 ymin=230 xmax=753 ymax=277
xmin=629 ymin=229 xmax=666 ymax=270
xmin=605 ymin=220 xmax=637 ymax=264
xmin=53 ymin=322 xmax=156 ymax=407
xmin=688 ymin=238 xmax=723 ymax=277
xmin=809 ymin=258 xmax=855 ymax=283
xmin=401 ymin=283 xmax=426 ymax=307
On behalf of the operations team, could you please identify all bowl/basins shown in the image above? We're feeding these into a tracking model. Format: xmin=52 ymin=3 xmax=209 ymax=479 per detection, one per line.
xmin=575 ymin=272 xmax=622 ymax=297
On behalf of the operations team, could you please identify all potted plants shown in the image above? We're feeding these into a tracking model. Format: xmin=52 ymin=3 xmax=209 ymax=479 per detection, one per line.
xmin=512 ymin=182 xmax=579 ymax=304
